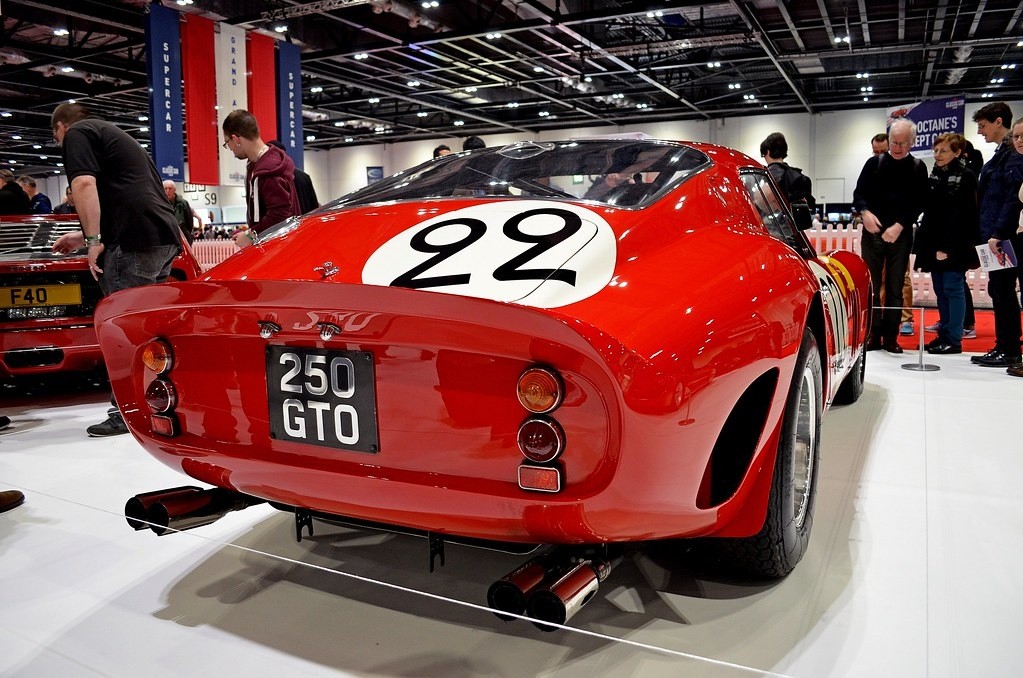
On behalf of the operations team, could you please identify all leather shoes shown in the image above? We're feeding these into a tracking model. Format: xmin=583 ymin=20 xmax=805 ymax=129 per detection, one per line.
xmin=971 ymin=346 xmax=1001 ymax=364
xmin=1006 ymin=365 xmax=1023 ymax=377
xmin=917 ymin=337 xmax=939 ymax=350
xmin=980 ymin=352 xmax=1022 ymax=366
xmin=866 ymin=336 xmax=882 ymax=350
xmin=883 ymin=338 xmax=903 ymax=353
xmin=926 ymin=341 xmax=962 ymax=354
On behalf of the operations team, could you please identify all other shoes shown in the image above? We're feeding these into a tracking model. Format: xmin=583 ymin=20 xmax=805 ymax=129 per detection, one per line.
xmin=0 ymin=416 xmax=11 ymax=430
xmin=0 ymin=490 xmax=25 ymax=513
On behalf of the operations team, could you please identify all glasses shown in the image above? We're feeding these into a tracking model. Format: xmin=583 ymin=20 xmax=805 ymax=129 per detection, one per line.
xmin=223 ymin=135 xmax=240 ymax=150
xmin=1012 ymin=134 xmax=1023 ymax=141
xmin=889 ymin=142 xmax=909 ymax=148
xmin=52 ymin=129 xmax=62 ymax=147
xmin=979 ymin=121 xmax=988 ymax=130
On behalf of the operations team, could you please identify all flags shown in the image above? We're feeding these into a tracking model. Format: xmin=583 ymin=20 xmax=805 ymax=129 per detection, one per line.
xmin=886 ymin=94 xmax=966 ymax=152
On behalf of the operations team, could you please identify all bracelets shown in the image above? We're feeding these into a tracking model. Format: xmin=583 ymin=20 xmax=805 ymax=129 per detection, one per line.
xmin=86 ymin=233 xmax=101 ymax=248
xmin=245 ymin=230 xmax=253 ymax=240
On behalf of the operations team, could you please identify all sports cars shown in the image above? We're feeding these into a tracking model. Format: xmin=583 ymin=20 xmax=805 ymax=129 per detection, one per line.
xmin=0 ymin=213 xmax=203 ymax=399
xmin=94 ymin=138 xmax=876 ymax=632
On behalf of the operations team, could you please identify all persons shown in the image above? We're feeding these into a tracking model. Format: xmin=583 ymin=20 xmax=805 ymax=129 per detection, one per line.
xmin=0 ymin=169 xmax=31 ymax=216
xmin=853 ymin=117 xmax=929 ymax=354
xmin=754 ymin=132 xmax=811 ymax=232
xmin=972 ymin=101 xmax=1023 ymax=367
xmin=1006 ymin=117 xmax=1023 ymax=377
xmin=433 ymin=144 xmax=451 ymax=158
xmin=15 ymin=175 xmax=52 ymax=214
xmin=50 ymin=103 xmax=183 ymax=437
xmin=162 ymin=180 xmax=193 ymax=246
xmin=54 ymin=185 xmax=77 ymax=214
xmin=871 ymin=134 xmax=915 ymax=336
xmin=222 ymin=109 xmax=301 ymax=250
xmin=266 ymin=140 xmax=319 ymax=215
xmin=923 ymin=140 xmax=984 ymax=339
xmin=912 ymin=134 xmax=981 ymax=355
xmin=462 ymin=137 xmax=486 ymax=152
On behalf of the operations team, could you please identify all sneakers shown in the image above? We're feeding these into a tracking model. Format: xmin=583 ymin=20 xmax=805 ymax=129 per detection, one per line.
xmin=900 ymin=321 xmax=915 ymax=335
xmin=924 ymin=319 xmax=942 ymax=332
xmin=962 ymin=326 xmax=976 ymax=339
xmin=87 ymin=408 xmax=130 ymax=437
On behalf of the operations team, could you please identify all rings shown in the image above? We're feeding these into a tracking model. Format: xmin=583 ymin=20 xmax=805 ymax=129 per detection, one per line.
xmin=90 ymin=266 xmax=92 ymax=268
